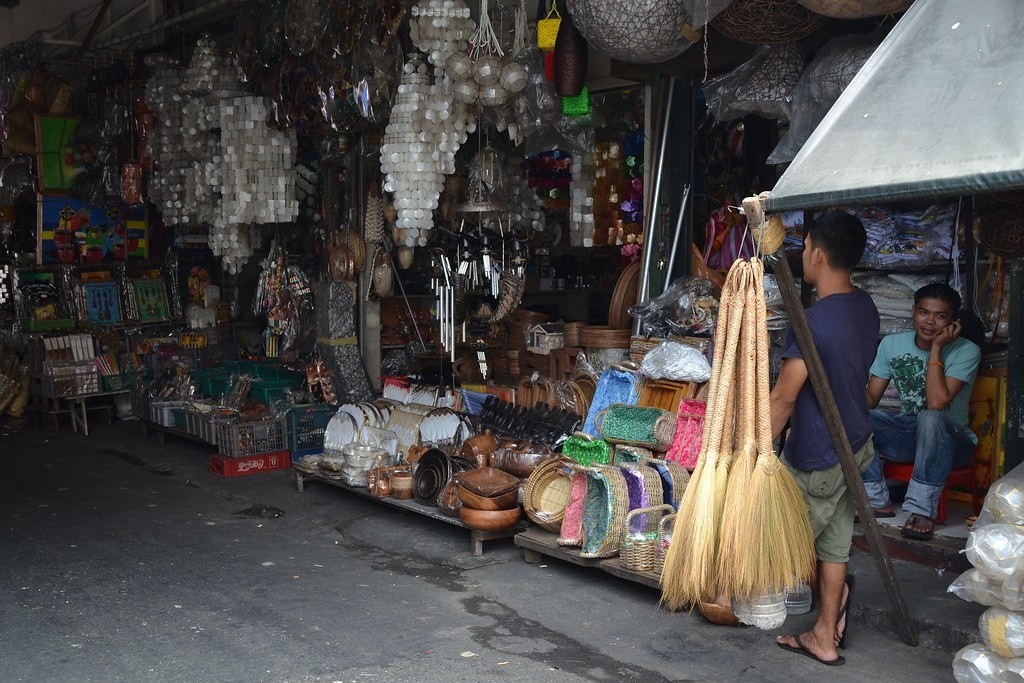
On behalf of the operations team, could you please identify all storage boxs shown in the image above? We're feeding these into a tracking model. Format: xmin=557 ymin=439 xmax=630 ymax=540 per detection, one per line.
xmin=34 ymin=335 xmax=340 ymax=459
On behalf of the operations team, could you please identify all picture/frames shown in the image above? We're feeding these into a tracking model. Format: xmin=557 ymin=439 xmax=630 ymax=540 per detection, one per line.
xmin=74 ymin=261 xmax=174 ymax=330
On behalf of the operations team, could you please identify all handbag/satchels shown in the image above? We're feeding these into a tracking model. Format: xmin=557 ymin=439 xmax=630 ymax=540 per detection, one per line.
xmin=690 ymin=242 xmax=728 ymax=289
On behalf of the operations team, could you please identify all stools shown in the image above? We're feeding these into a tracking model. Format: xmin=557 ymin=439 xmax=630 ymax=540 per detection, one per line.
xmin=883 ymin=460 xmax=980 ymax=520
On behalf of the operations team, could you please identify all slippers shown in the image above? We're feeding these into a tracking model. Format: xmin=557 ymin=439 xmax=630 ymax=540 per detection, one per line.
xmin=777 ymin=635 xmax=846 ymax=666
xmin=900 ymin=517 xmax=935 ymax=540
xmin=833 ymin=574 xmax=855 ymax=649
xmin=855 ymin=509 xmax=895 ymax=520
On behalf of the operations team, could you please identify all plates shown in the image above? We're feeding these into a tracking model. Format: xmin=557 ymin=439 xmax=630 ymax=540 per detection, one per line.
xmin=413 ymin=448 xmax=453 ymax=506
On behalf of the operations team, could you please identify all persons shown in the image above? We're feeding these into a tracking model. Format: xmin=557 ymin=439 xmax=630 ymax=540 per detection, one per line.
xmin=853 ymin=284 xmax=979 ymax=540
xmin=769 ymin=210 xmax=881 ymax=666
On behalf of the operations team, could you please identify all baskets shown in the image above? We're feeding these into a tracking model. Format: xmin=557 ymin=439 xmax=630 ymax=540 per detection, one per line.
xmin=630 ymin=334 xmax=713 ymax=364
xmin=517 ymin=373 xmax=599 ymax=430
xmin=665 ymin=395 xmax=707 ymax=470
xmin=613 ymin=444 xmax=653 ymax=466
xmin=594 ymin=403 xmax=677 ymax=451
xmin=583 ymin=363 xmax=644 ymax=439
xmin=562 ymin=432 xmax=612 ymax=465
xmin=524 ymin=456 xmax=689 ymax=574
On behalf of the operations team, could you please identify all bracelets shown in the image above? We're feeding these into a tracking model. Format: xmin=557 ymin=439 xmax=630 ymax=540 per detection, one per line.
xmin=928 ymin=361 xmax=942 ymax=366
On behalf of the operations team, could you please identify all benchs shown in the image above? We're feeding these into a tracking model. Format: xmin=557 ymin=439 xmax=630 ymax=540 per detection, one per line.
xmin=65 ymin=389 xmax=131 ymax=435
